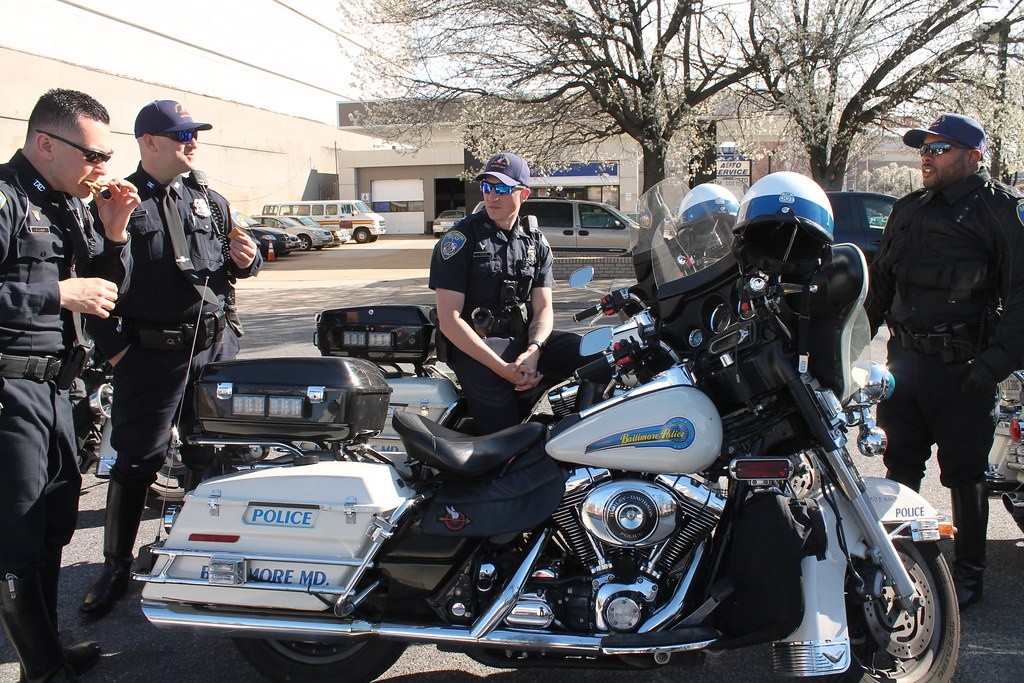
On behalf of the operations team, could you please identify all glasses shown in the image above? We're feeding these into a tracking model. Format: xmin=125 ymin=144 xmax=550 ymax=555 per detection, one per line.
xmin=480 ymin=181 xmax=516 ymax=195
xmin=36 ymin=130 xmax=112 ymax=164
xmin=153 ymin=131 xmax=197 ymax=142
xmin=920 ymin=141 xmax=968 ymax=157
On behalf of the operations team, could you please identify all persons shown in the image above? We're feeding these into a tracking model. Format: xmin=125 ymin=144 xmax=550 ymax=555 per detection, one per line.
xmin=429 ymin=153 xmax=614 ymax=436
xmin=862 ymin=112 xmax=1024 ymax=610
xmin=77 ymin=100 xmax=264 ymax=626
xmin=0 ymin=88 xmax=141 ymax=683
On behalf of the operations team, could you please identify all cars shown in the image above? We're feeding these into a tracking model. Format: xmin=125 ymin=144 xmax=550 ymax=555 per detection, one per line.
xmin=254 ymin=216 xmax=334 ymax=251
xmin=823 ymin=192 xmax=901 ymax=257
xmin=432 ymin=209 xmax=466 ymax=238
xmin=233 ymin=212 xmax=303 ymax=258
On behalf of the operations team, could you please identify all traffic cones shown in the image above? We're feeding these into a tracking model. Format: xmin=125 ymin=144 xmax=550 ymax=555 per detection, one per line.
xmin=267 ymin=239 xmax=276 ymax=262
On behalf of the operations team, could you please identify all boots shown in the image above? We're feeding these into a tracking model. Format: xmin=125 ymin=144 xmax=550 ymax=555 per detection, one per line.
xmin=951 ymin=477 xmax=989 ymax=610
xmin=0 ymin=550 xmax=101 ymax=683
xmin=81 ymin=475 xmax=146 ymax=620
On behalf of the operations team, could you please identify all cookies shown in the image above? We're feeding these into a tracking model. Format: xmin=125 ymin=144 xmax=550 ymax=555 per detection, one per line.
xmin=229 ymin=226 xmax=244 ymax=238
xmin=84 ymin=181 xmax=102 ymax=195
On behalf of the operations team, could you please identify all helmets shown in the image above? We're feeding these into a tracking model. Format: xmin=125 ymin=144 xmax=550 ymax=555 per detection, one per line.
xmin=678 ymin=183 xmax=738 ymax=222
xmin=732 ymin=172 xmax=834 ymax=242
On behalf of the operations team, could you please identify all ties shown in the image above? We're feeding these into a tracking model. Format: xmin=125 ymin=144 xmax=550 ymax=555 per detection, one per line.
xmin=162 ymin=184 xmax=220 ymax=305
xmin=71 ymin=255 xmax=88 ymax=348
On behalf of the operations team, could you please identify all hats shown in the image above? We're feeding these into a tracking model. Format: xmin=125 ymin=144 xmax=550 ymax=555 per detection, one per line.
xmin=135 ymin=100 xmax=212 ymax=138
xmin=476 ymin=154 xmax=530 ymax=188
xmin=904 ymin=113 xmax=986 ymax=160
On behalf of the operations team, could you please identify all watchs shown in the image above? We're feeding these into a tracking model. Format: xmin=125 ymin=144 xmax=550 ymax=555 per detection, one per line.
xmin=526 ymin=341 xmax=546 ymax=359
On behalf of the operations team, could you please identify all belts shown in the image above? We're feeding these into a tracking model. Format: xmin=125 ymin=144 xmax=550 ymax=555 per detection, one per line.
xmin=896 ymin=322 xmax=968 ymax=351
xmin=0 ymin=355 xmax=68 ymax=380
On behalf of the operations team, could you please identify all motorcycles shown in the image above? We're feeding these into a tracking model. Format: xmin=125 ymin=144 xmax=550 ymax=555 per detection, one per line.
xmin=81 ymin=168 xmax=1023 ymax=683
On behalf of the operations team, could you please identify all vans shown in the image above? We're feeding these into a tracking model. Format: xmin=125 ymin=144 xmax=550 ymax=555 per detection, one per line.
xmin=262 ymin=200 xmax=389 ymax=244
xmin=471 ymin=196 xmax=639 ymax=255
